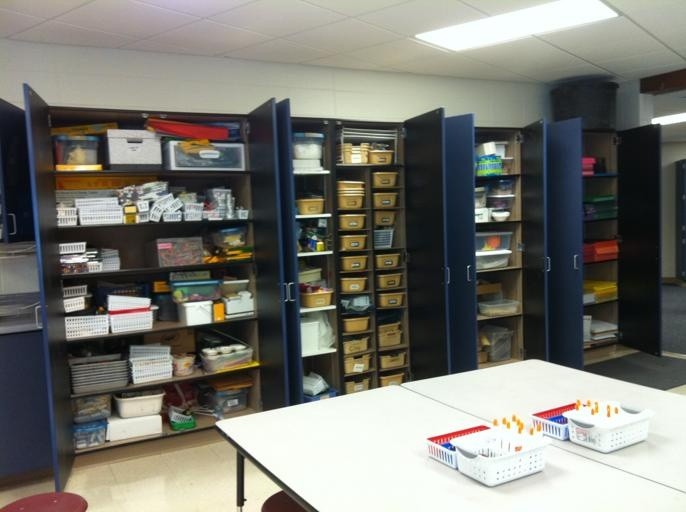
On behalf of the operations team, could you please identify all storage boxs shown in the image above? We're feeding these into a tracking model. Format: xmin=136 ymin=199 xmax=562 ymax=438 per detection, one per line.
xmin=337 ymin=141 xmax=370 ymax=165
xmin=340 ymin=236 xmax=369 ymax=251
xmin=426 ymin=424 xmax=490 ymax=469
xmin=375 ymin=212 xmax=397 ymax=227
xmin=475 ymin=208 xmax=488 ymax=223
xmin=338 ymin=215 xmax=368 ymax=229
xmin=483 ymin=178 xmax=515 ymax=195
xmin=56 ymin=134 xmax=100 ymax=168
xmin=296 ymin=197 xmax=325 ymax=215
xmin=294 ymin=134 xmax=325 ymax=160
xmin=342 ymin=354 xmax=374 ymax=372
xmin=340 ymin=278 xmax=369 ymax=292
xmin=369 ymin=150 xmax=394 ymax=163
xmin=63 ymin=314 xmax=109 ymax=341
xmin=342 ymin=317 xmax=371 ymax=331
xmin=376 ymin=322 xmax=404 ymax=347
xmin=478 ymin=298 xmax=521 ymax=315
xmin=222 ymin=289 xmax=255 ymax=318
xmin=156 ymin=293 xmax=179 ymax=321
xmin=375 ymin=253 xmax=401 ymax=270
xmin=113 ymin=389 xmax=166 ymax=418
xmin=378 ymin=370 xmax=405 ymax=387
xmin=450 ymin=418 xmax=553 ymax=487
xmin=69 ymin=396 xmax=112 ymax=422
xmin=142 ymin=237 xmax=203 ymax=265
xmin=200 ymin=348 xmax=254 ymax=371
xmin=485 ymin=327 xmax=516 ymax=361
xmin=140 ymin=328 xmax=195 ymax=352
xmin=489 ymin=207 xmax=513 ymax=221
xmin=496 ymin=141 xmax=509 ymax=157
xmin=475 ymin=186 xmax=488 ymax=207
xmin=372 ymin=172 xmax=399 ymax=186
xmin=339 ymin=255 xmax=369 ymax=272
xmin=196 ymin=386 xmax=250 ymax=414
xmin=376 ymin=273 xmax=404 ymax=289
xmin=170 ymin=281 xmax=221 ymax=300
xmin=503 ymin=157 xmax=515 ymax=173
xmin=300 ymin=319 xmax=321 ymax=355
xmin=490 ymin=195 xmax=516 ymax=207
xmin=475 ymin=233 xmax=514 ymax=252
xmin=345 ymin=377 xmax=370 ymax=393
xmin=337 ymin=179 xmax=366 ymax=210
xmin=107 ymin=129 xmax=163 ymax=167
xmin=343 ymin=335 xmax=370 ymax=351
xmin=108 ymin=307 xmax=160 ymax=333
xmin=584 ymin=277 xmax=619 ymax=302
xmin=73 ymin=423 xmax=107 ymax=449
xmin=475 ymin=250 xmax=514 ymax=270
xmin=476 ymin=155 xmax=502 ymax=175
xmin=302 ymin=287 xmax=335 ymax=307
xmin=178 ymin=300 xmax=214 ymax=327
xmin=380 ymin=352 xmax=406 ymax=367
xmin=531 ymin=401 xmax=582 ymax=441
xmin=584 ymin=240 xmax=619 ymax=261
xmin=378 ymin=293 xmax=407 ymax=308
xmin=561 ymin=399 xmax=658 ymax=454
xmin=106 ymin=418 xmax=163 ymax=442
xmin=374 ymin=230 xmax=397 ymax=249
xmin=168 ymin=138 xmax=245 ymax=172
xmin=372 ymin=193 xmax=400 ymax=209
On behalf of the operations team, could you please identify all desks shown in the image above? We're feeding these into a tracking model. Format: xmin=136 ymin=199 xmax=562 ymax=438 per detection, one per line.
xmin=215 ymin=384 xmax=686 ymax=511
xmin=403 ymin=357 xmax=686 ymax=494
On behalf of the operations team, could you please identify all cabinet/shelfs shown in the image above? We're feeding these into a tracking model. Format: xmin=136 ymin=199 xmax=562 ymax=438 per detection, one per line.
xmin=0 ymin=89 xmax=47 ymax=486
xmin=549 ymin=119 xmax=661 ymax=373
xmin=448 ymin=114 xmax=556 ymax=374
xmin=15 ymin=78 xmax=293 ymax=493
xmin=278 ymin=97 xmax=461 ymax=410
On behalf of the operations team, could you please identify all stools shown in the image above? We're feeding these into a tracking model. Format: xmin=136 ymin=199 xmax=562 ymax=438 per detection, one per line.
xmin=2 ymin=490 xmax=86 ymax=512
